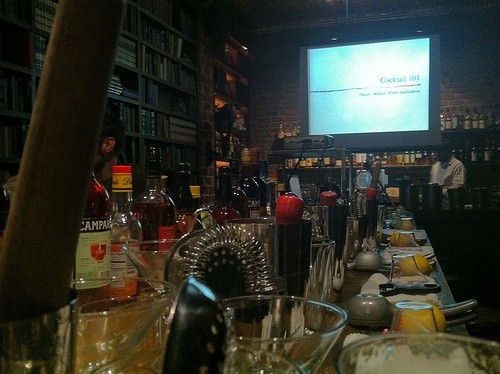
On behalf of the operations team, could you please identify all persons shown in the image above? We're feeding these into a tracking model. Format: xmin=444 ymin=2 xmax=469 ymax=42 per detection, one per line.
xmin=92 ymin=110 xmax=146 ymax=201
xmin=425 ymin=136 xmax=467 ymax=200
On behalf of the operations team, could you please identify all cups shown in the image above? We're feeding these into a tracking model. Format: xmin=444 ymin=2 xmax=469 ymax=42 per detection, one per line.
xmin=0 ymin=287 xmax=80 ymax=374
xmin=89 ymin=344 xmax=306 ymax=374
xmin=72 ymin=275 xmax=179 ymax=374
xmin=220 ymin=295 xmax=348 ymax=374
xmin=311 ymin=235 xmax=336 ymax=303
xmin=335 ymin=331 xmax=500 ymax=374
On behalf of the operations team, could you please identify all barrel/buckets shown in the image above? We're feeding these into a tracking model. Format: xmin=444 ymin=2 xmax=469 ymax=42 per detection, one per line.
xmin=224 ymin=217 xmax=312 ymax=311
xmin=398 ymin=183 xmax=489 ymax=210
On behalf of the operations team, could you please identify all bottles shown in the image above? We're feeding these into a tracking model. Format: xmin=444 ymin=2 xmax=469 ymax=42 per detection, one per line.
xmin=241 ymin=146 xmax=286 ymax=219
xmin=110 ymin=165 xmax=144 ymax=306
xmin=132 ymin=146 xmax=178 ymax=283
xmin=276 ymin=122 xmax=301 ymax=140
xmin=215 ymin=131 xmax=241 ymax=156
xmin=70 ymin=173 xmax=114 ymax=313
xmin=189 ymin=184 xmax=204 ymax=231
xmin=320 ymin=190 xmax=342 ymax=260
xmin=357 ymin=188 xmax=378 ymax=251
xmin=212 ymin=165 xmax=242 ymax=226
xmin=439 ymin=107 xmax=500 ymax=129
xmin=174 ymin=161 xmax=203 ymax=239
xmin=299 ymin=146 xmax=500 ymax=167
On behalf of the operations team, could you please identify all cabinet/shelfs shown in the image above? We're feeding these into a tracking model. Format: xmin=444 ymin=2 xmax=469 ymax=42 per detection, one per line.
xmin=36 ymin=2 xmax=199 ymax=175
xmin=277 ymin=126 xmax=500 ymax=171
xmin=212 ymin=34 xmax=251 ymax=163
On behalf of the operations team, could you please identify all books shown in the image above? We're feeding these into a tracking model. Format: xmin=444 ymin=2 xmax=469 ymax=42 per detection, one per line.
xmin=143 ymin=21 xmax=196 ymax=65
xmin=117 ymin=102 xmax=135 ymax=132
xmin=140 ymin=77 xmax=197 ymax=116
xmin=141 ymin=45 xmax=197 ymax=93
xmin=137 ymin=0 xmax=197 ymax=42
xmin=0 ymin=0 xmax=58 ymax=34
xmin=0 ymin=70 xmax=32 ymax=113
xmin=114 ymin=6 xmax=137 ymax=68
xmin=0 ymin=121 xmax=29 ymax=187
xmin=34 ymin=35 xmax=47 ymax=70
xmin=144 ymin=144 xmax=197 ymax=169
xmin=107 ymin=74 xmax=138 ymax=100
xmin=141 ymin=110 xmax=197 ymax=144
